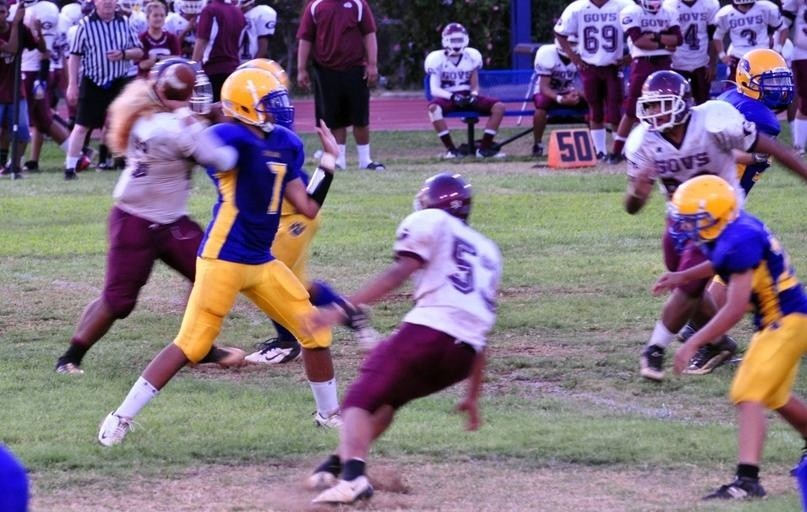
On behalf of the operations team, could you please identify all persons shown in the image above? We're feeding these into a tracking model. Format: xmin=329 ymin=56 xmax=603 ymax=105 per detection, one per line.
xmin=98 ymin=69 xmax=341 ymax=449
xmin=424 ymin=22 xmax=506 ymax=159
xmin=652 ymin=173 xmax=807 ymax=501
xmin=295 ymin=0 xmax=385 ymax=170
xmin=232 ymin=59 xmax=386 ymax=363
xmin=52 ymin=56 xmax=245 ymax=376
xmin=553 ymin=0 xmax=806 ymax=164
xmin=529 ymin=38 xmax=591 ymax=156
xmin=305 ymin=173 xmax=502 ymax=505
xmin=0 ymin=1 xmax=281 ymax=180
xmin=624 ymin=72 xmax=807 ymax=382
xmin=674 ymin=49 xmax=796 ymax=341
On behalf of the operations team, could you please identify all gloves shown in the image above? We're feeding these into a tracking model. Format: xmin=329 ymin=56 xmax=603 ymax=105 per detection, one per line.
xmin=452 ymin=91 xmax=477 ymax=108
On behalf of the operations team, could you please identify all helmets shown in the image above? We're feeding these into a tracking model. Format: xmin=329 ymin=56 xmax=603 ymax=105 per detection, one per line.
xmin=736 ymin=48 xmax=794 ymax=114
xmin=441 ymin=22 xmax=470 ymax=57
xmin=149 ymin=58 xmax=294 ymax=134
xmin=553 ymin=22 xmax=579 ymax=56
xmin=635 ymin=69 xmax=692 ymax=132
xmin=413 ymin=171 xmax=470 ymax=223
xmin=662 ymin=172 xmax=740 ymax=254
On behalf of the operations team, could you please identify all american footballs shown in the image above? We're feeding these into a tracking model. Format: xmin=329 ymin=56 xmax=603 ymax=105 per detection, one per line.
xmin=157 ymin=63 xmax=196 ymax=101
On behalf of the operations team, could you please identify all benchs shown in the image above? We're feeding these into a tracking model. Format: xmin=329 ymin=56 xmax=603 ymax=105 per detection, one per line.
xmin=423 ymin=71 xmax=632 ymax=159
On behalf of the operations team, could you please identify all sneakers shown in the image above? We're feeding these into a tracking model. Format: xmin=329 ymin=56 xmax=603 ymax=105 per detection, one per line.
xmin=639 ymin=314 xmax=807 ymax=501
xmin=335 ymin=144 xmax=623 ymax=172
xmin=0 ymin=147 xmax=125 ymax=182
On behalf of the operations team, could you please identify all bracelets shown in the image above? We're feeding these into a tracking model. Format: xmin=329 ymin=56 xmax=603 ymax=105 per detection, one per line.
xmin=469 ymin=91 xmax=478 ymax=96
